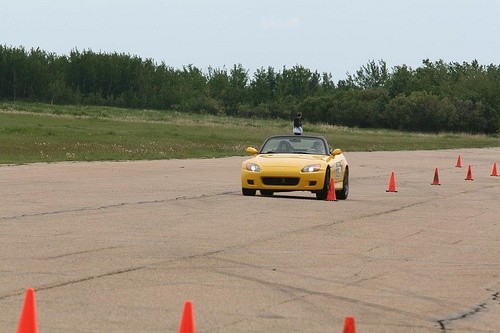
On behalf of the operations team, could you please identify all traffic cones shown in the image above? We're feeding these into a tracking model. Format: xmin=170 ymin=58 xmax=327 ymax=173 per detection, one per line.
xmin=179 ymin=300 xmax=195 ymax=333
xmin=341 ymin=316 xmax=356 ymax=333
xmin=430 ymin=167 xmax=442 ymax=185
xmin=15 ymin=287 xmax=38 ymax=333
xmin=464 ymin=164 xmax=474 ymax=180
xmin=325 ymin=178 xmax=337 ymax=201
xmin=454 ymin=155 xmax=462 ymax=167
xmin=490 ymin=163 xmax=498 ymax=176
xmin=385 ymin=171 xmax=398 ymax=193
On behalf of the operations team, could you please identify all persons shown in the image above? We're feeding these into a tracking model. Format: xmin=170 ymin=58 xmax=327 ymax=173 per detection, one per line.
xmin=311 ymin=140 xmax=323 ymax=154
xmin=293 ymin=112 xmax=303 ymax=142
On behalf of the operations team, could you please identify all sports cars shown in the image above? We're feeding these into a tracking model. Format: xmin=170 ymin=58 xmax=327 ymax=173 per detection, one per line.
xmin=240 ymin=135 xmax=350 ymax=201
